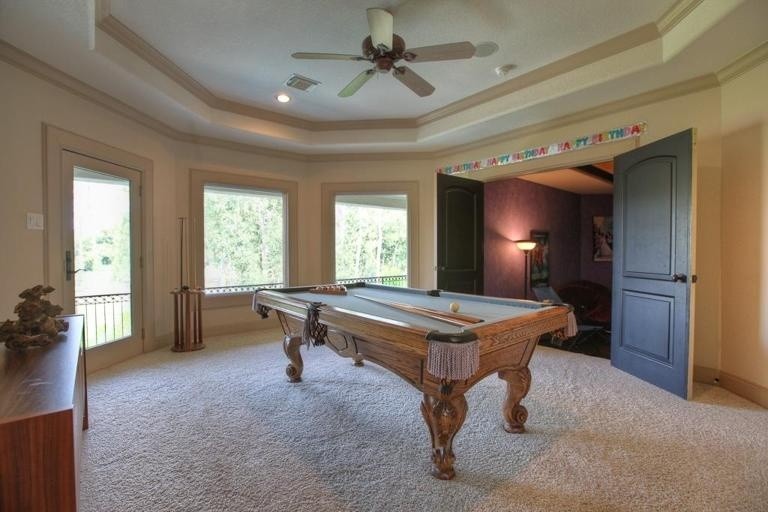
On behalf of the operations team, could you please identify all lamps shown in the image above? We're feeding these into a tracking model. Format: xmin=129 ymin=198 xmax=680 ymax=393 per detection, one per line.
xmin=514 ymin=240 xmax=537 ymax=301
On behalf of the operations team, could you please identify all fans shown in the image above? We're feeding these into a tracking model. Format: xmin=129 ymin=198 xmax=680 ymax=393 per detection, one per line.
xmin=292 ymin=7 xmax=476 ymax=98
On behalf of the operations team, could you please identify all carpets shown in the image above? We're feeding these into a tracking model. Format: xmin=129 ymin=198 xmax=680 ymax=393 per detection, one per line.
xmin=75 ymin=328 xmax=768 ymax=512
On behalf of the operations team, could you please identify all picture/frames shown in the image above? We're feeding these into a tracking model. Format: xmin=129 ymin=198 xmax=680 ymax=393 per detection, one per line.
xmin=591 ymin=213 xmax=614 ymax=263
xmin=529 ymin=229 xmax=551 ymax=293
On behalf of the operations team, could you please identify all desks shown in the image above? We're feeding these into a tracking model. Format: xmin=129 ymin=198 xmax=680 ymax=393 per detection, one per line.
xmin=252 ymin=279 xmax=578 ymax=479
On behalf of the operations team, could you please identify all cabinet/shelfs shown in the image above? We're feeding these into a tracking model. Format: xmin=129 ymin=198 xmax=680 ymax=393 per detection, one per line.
xmin=0 ymin=311 xmax=88 ymax=511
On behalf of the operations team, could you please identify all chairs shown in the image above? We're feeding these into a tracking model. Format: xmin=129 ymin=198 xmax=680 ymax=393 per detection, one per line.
xmin=547 ymin=280 xmax=615 ymax=351
